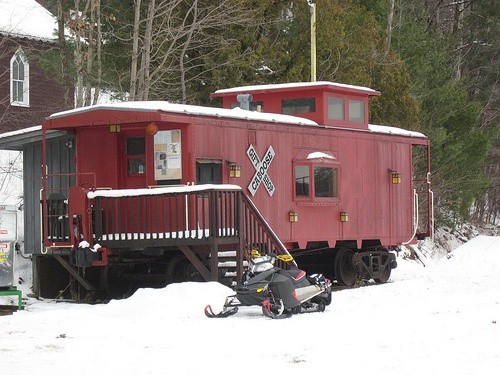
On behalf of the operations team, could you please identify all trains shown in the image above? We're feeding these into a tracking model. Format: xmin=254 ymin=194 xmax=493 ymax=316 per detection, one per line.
xmin=42 ymin=81 xmax=431 ymax=294
